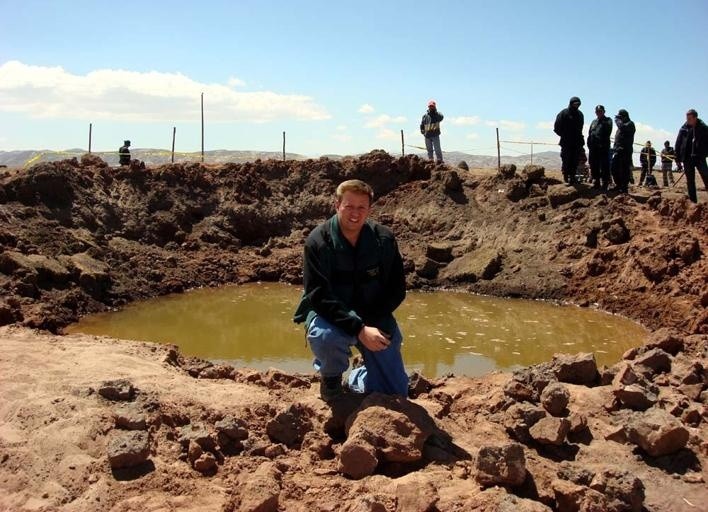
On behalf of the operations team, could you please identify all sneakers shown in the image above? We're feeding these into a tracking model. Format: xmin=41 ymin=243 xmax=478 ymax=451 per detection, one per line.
xmin=319 ymin=372 xmax=343 ymax=402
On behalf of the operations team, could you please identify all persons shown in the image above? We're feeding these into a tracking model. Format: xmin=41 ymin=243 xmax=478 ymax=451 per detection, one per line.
xmin=636 ymin=141 xmax=656 ymax=187
xmin=553 ymin=97 xmax=587 ymax=186
xmin=118 ymin=140 xmax=131 ymax=168
xmin=420 ymin=100 xmax=444 ymax=165
xmin=607 ymin=109 xmax=635 ymax=197
xmin=660 ymin=141 xmax=675 ymax=188
xmin=675 ymin=109 xmax=708 ymax=203
xmin=584 ymin=105 xmax=612 ymax=195
xmin=293 ymin=180 xmax=410 ymax=402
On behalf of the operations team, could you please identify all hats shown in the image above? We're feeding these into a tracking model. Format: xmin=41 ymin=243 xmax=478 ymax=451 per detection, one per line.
xmin=428 ymin=101 xmax=435 ymax=107
xmin=616 ymin=110 xmax=628 ymax=116
